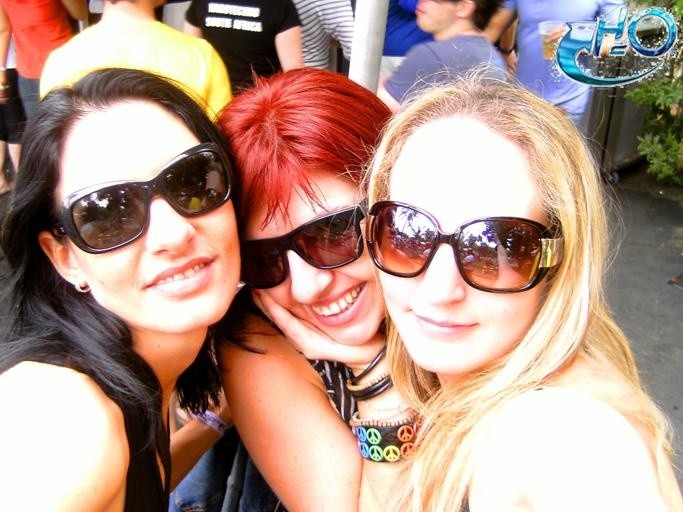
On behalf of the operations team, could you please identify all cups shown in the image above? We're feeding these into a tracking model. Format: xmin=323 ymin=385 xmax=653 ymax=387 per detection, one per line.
xmin=538 ymin=21 xmax=567 ymax=60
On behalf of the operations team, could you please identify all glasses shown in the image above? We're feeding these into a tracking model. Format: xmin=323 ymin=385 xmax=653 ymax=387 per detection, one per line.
xmin=52 ymin=143 xmax=233 ymax=253
xmin=240 ymin=197 xmax=368 ymax=288
xmin=369 ymin=201 xmax=564 ymax=294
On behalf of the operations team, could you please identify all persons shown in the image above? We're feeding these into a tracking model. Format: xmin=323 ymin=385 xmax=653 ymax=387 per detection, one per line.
xmin=0 ymin=2 xmax=682 ymax=511
